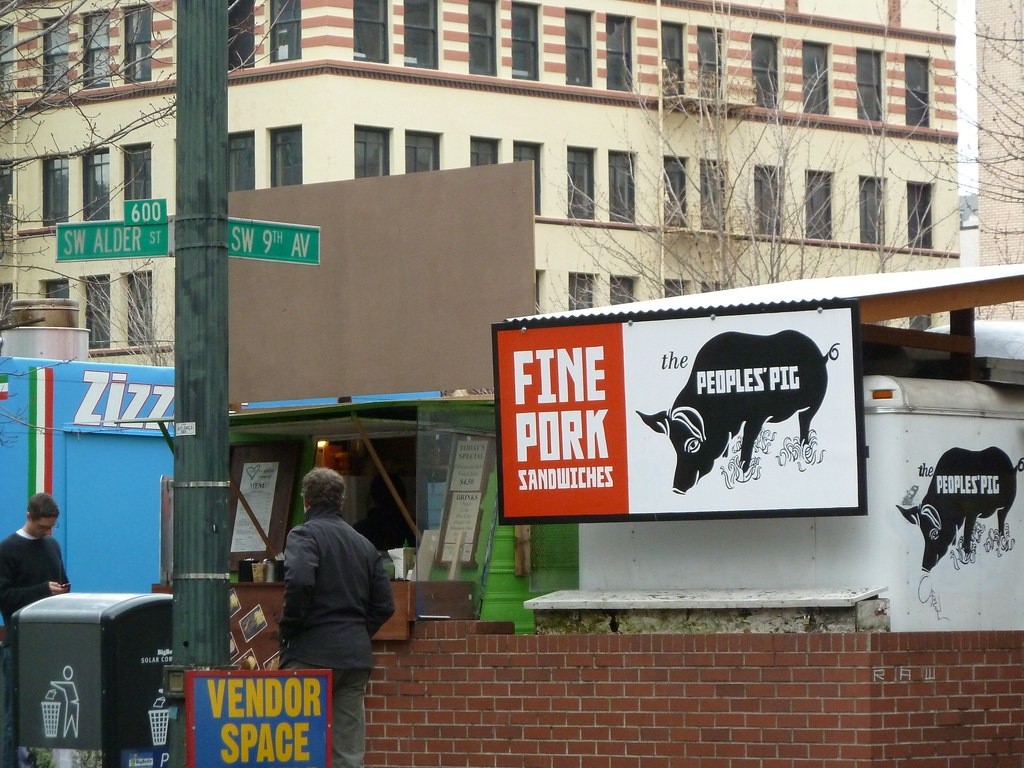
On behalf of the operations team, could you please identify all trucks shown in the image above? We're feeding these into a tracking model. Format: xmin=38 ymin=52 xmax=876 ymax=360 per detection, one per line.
xmin=0 ymin=358 xmax=446 ymax=629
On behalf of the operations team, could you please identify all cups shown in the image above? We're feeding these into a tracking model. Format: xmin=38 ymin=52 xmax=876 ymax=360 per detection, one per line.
xmin=238 ymin=559 xmax=285 ymax=581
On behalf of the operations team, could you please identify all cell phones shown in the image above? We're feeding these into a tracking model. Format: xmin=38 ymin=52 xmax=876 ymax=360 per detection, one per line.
xmin=60 ymin=583 xmax=72 ymax=588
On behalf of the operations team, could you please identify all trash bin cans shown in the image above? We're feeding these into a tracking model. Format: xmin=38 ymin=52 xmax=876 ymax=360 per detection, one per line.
xmin=10 ymin=592 xmax=173 ymax=767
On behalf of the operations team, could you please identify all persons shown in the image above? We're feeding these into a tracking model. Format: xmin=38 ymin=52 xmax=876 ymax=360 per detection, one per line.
xmin=278 ymin=466 xmax=395 ymax=768
xmin=0 ymin=492 xmax=75 ymax=768
xmin=354 ymin=475 xmax=415 ymax=551
